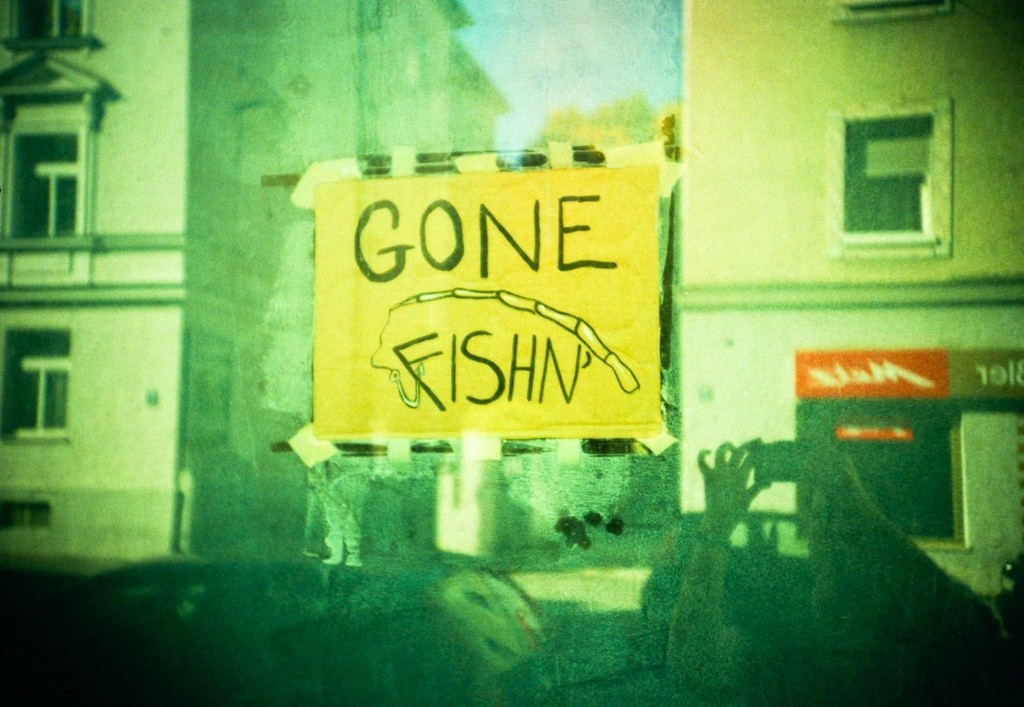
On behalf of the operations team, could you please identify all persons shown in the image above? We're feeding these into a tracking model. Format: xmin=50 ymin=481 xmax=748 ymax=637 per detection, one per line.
xmin=657 ymin=430 xmax=1014 ymax=707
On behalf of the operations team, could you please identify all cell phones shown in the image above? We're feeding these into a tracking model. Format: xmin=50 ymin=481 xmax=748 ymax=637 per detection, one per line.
xmin=756 ymin=439 xmax=836 ymax=484
